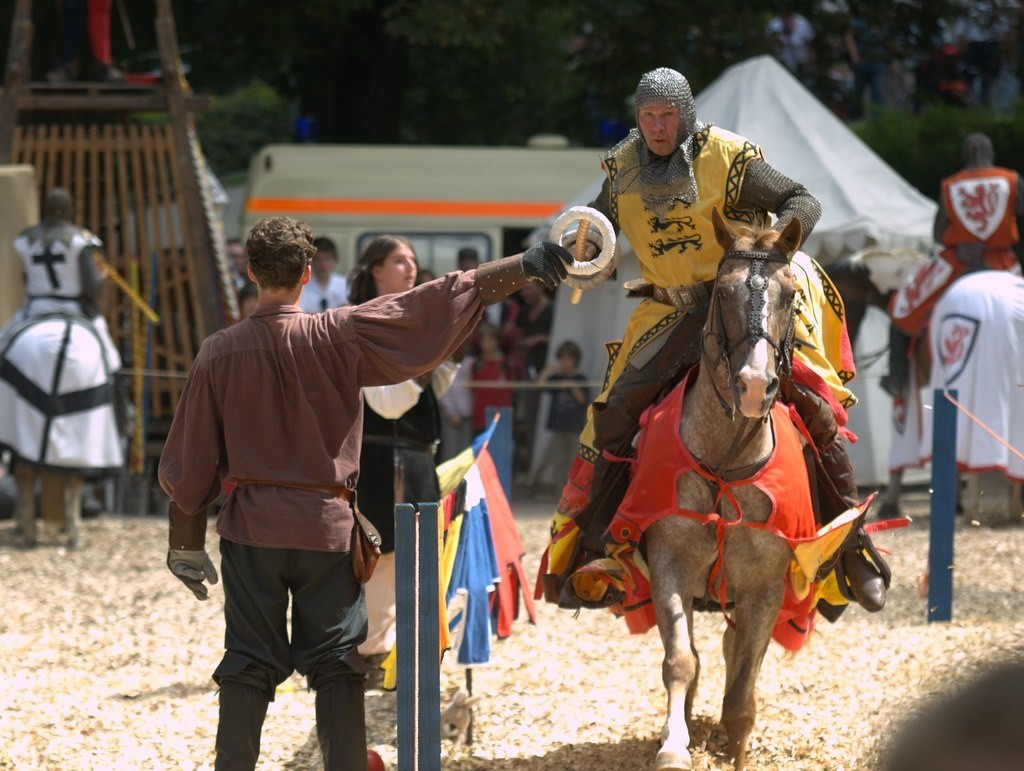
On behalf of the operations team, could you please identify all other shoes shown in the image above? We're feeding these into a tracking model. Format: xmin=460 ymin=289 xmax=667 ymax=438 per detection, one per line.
xmin=367 ymin=749 xmax=385 ymax=771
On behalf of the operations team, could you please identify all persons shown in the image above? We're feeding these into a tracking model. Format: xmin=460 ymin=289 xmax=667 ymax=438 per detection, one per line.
xmin=763 ymin=0 xmax=1024 ymax=121
xmin=557 ymin=66 xmax=889 ymax=613
xmin=0 ymin=184 xmax=138 ymax=439
xmin=878 ymin=132 xmax=1024 ymax=398
xmin=335 ymin=234 xmax=473 ymax=690
xmin=226 ymin=238 xmax=593 ymax=513
xmin=157 ymin=215 xmax=575 ymax=771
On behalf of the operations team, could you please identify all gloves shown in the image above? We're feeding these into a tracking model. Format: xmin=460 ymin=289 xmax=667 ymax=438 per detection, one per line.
xmin=520 ymin=241 xmax=576 ymax=292
xmin=166 ymin=549 xmax=218 ymax=601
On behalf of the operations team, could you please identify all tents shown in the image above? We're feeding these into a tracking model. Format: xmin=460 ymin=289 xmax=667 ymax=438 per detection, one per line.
xmin=524 ymin=52 xmax=942 ymax=486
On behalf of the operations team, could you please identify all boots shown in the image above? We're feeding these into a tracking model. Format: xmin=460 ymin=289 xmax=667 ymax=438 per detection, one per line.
xmin=808 ymin=435 xmax=886 ymax=612
xmin=557 ymin=400 xmax=632 ymax=608
xmin=879 ymin=323 xmax=912 ymax=397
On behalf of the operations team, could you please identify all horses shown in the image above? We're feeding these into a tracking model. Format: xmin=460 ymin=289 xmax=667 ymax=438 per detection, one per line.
xmin=639 ymin=201 xmax=810 ymax=771
xmin=819 ymin=235 xmax=1024 ymax=528
xmin=0 ymin=308 xmax=119 ymax=550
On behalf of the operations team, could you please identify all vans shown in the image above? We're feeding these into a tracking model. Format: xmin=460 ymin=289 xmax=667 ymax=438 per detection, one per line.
xmin=237 ymin=133 xmax=614 ymax=372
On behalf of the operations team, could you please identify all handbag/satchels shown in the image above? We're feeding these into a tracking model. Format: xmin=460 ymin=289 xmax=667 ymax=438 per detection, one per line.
xmin=233 ymin=477 xmax=382 ymax=584
